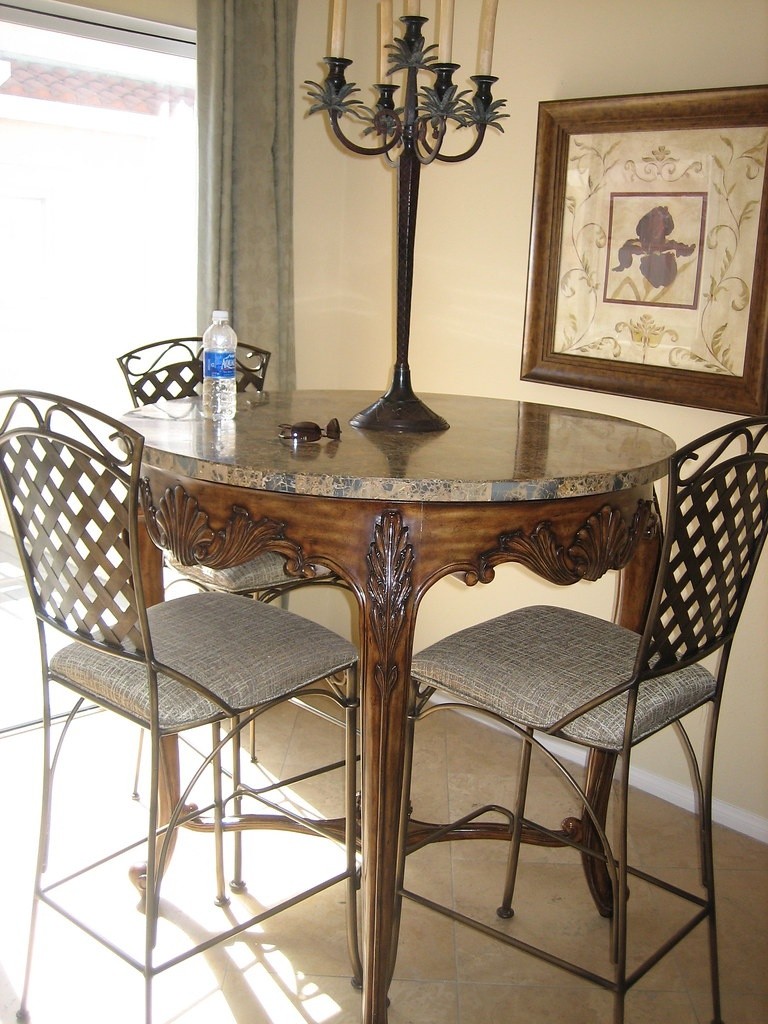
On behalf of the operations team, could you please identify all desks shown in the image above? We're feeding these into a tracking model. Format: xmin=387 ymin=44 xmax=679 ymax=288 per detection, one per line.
xmin=112 ymin=384 xmax=677 ymax=1024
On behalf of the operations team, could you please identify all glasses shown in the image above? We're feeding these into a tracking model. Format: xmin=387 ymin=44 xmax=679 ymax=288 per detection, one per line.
xmin=277 ymin=418 xmax=342 ymax=443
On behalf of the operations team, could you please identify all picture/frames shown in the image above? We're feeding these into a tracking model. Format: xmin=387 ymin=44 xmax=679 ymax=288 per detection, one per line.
xmin=518 ymin=84 xmax=768 ymax=418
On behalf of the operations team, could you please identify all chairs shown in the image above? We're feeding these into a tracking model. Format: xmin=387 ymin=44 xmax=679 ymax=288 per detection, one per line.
xmin=0 ymin=388 xmax=365 ymax=1024
xmin=117 ymin=336 xmax=361 ymax=805
xmin=384 ymin=416 xmax=768 ymax=1024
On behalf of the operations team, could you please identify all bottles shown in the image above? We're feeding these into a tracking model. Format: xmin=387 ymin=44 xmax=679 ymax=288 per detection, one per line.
xmin=202 ymin=310 xmax=238 ymax=420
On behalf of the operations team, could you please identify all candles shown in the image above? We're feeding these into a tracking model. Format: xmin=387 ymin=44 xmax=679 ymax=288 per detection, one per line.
xmin=378 ymin=0 xmax=393 ymax=86
xmin=437 ymin=0 xmax=455 ymax=64
xmin=474 ymin=0 xmax=499 ymax=76
xmin=407 ymin=0 xmax=421 ymax=16
xmin=328 ymin=0 xmax=348 ymax=58
